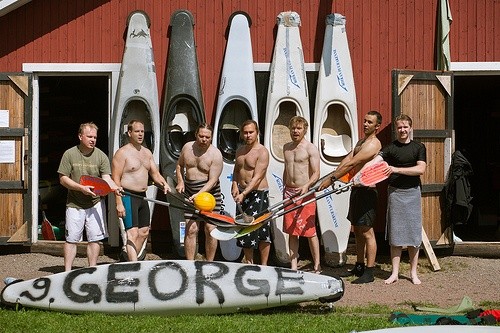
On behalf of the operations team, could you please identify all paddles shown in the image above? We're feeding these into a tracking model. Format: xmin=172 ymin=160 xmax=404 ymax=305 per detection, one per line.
xmin=79 ymin=175 xmax=235 ymax=225
xmin=210 ymin=160 xmax=392 ymax=241
xmin=148 ymin=177 xmax=236 ymax=227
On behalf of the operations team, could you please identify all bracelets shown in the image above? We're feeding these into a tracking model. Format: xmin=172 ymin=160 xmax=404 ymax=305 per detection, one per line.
xmin=242 ymin=192 xmax=246 ymax=198
xmin=330 ymin=172 xmax=336 ymax=183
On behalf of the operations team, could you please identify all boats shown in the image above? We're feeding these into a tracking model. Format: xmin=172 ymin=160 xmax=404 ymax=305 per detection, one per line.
xmin=159 ymin=7 xmax=207 ymax=259
xmin=109 ymin=9 xmax=160 ymax=261
xmin=263 ymin=10 xmax=311 ymax=267
xmin=1 ymin=260 xmax=345 ymax=319
xmin=210 ymin=10 xmax=261 ymax=263
xmin=312 ymin=12 xmax=360 ymax=268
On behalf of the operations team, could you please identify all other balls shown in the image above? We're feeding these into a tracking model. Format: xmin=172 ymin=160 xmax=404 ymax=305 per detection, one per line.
xmin=194 ymin=192 xmax=216 ymax=211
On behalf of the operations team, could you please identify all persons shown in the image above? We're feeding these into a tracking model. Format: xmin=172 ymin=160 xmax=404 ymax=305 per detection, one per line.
xmin=317 ymin=111 xmax=383 ymax=285
xmin=230 ymin=120 xmax=272 ymax=267
xmin=110 ymin=119 xmax=171 ymax=261
xmin=350 ymin=114 xmax=428 ymax=285
xmin=57 ymin=123 xmax=125 ymax=272
xmin=282 ymin=116 xmax=323 ymax=275
xmin=176 ymin=122 xmax=223 ymax=261
